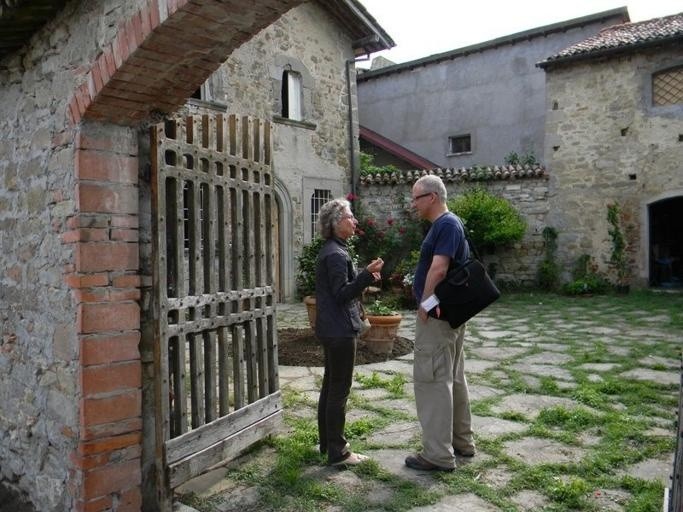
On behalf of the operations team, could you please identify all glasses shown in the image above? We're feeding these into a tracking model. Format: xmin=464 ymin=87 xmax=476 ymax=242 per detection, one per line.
xmin=411 ymin=192 xmax=437 ymax=202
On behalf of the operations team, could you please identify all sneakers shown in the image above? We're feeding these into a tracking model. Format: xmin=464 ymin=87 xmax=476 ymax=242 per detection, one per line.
xmin=405 ymin=454 xmax=455 ymax=472
xmin=332 ymin=443 xmax=369 ymax=466
xmin=452 ymin=444 xmax=474 ymax=457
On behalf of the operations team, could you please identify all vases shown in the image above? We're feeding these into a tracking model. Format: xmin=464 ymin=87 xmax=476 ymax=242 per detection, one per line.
xmin=304 ymin=294 xmax=316 ymax=332
xmin=366 ymin=335 xmax=395 ymax=354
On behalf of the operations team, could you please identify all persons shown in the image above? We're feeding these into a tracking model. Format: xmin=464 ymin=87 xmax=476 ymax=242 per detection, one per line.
xmin=312 ymin=197 xmax=385 ymax=467
xmin=403 ymin=174 xmax=477 ymax=473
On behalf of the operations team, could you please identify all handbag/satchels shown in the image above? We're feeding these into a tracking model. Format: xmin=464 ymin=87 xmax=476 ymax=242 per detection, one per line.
xmin=434 ymin=259 xmax=501 ymax=329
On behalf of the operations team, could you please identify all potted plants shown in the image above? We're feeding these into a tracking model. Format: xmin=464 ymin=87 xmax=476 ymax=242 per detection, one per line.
xmin=363 ymin=300 xmax=403 ymax=337
